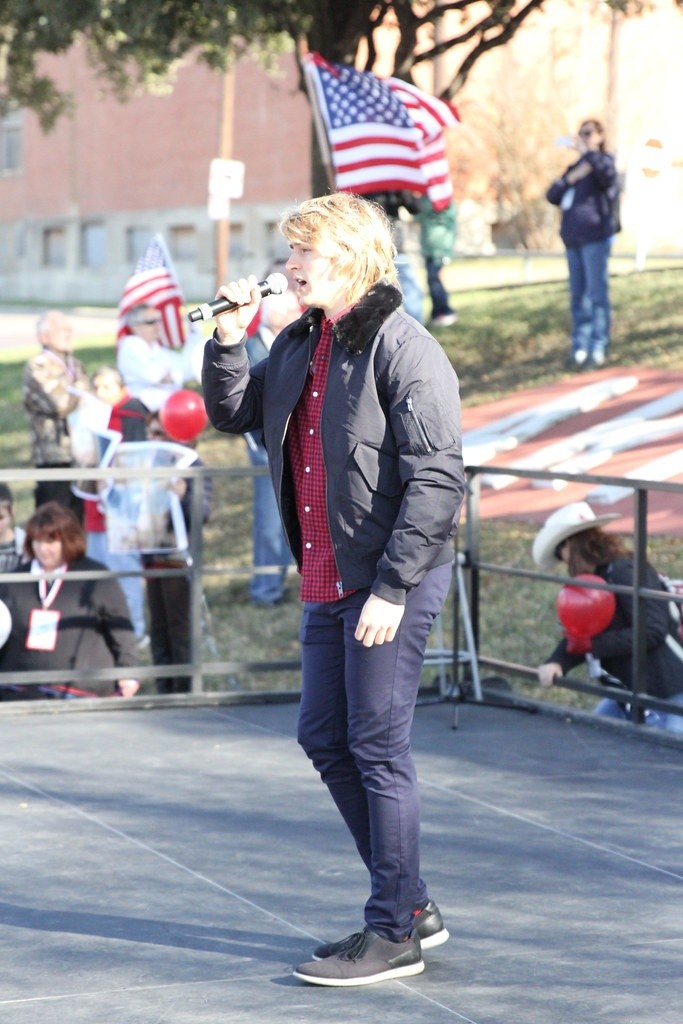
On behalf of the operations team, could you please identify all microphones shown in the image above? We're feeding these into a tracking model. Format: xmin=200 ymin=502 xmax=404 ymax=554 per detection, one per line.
xmin=188 ymin=273 xmax=289 ymax=323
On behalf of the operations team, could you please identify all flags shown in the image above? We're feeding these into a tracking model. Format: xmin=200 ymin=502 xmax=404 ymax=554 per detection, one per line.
xmin=313 ymin=58 xmax=462 ymax=212
xmin=116 ymin=239 xmax=185 ymax=348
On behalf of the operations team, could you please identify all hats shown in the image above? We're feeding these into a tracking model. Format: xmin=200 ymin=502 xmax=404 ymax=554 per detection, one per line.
xmin=531 ymin=502 xmax=623 ymax=569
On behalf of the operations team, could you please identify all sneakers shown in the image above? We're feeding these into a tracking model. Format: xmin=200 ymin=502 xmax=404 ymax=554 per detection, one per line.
xmin=312 ymin=901 xmax=449 ymax=960
xmin=293 ymin=929 xmax=425 ymax=986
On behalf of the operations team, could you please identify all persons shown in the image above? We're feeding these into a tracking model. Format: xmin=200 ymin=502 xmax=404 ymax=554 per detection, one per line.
xmin=532 ymin=500 xmax=683 ymax=733
xmin=0 ymin=482 xmax=142 ymax=704
xmin=118 ymin=303 xmax=204 ymax=417
xmin=202 ymin=194 xmax=465 ymax=986
xmin=76 ymin=368 xmax=212 ymax=694
xmin=409 ymin=189 xmax=459 ymax=327
xmin=23 ymin=311 xmax=85 ymax=521
xmin=546 ymin=119 xmax=622 ymax=369
xmin=243 ymin=288 xmax=296 ymax=605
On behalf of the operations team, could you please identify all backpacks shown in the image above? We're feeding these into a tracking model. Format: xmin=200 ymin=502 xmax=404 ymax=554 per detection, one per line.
xmin=657 ymin=571 xmax=683 ymax=642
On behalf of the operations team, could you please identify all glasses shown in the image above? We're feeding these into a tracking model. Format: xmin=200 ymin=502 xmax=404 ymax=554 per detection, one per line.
xmin=135 ymin=317 xmax=161 ymax=325
xmin=579 ymin=130 xmax=598 ymax=137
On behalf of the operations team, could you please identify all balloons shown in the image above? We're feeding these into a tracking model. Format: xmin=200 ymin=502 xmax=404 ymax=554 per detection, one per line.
xmin=161 ymin=390 xmax=209 ymax=440
xmin=556 ymin=573 xmax=615 ymax=640
